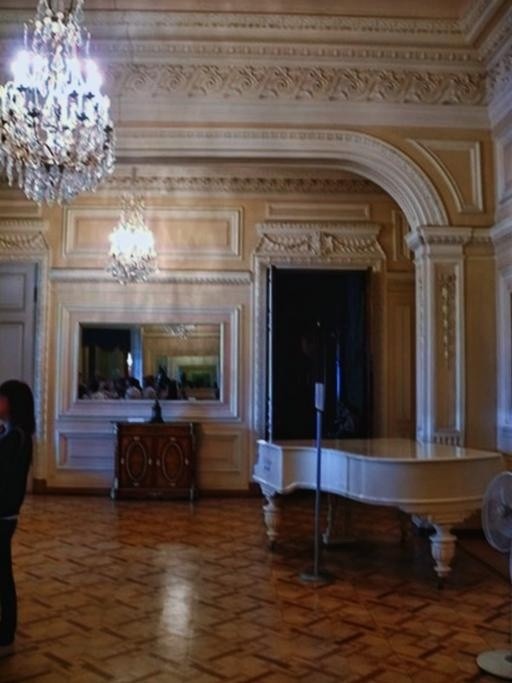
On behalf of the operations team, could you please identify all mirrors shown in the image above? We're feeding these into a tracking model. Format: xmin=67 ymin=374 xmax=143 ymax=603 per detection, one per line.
xmin=72 ymin=319 xmax=225 ymax=405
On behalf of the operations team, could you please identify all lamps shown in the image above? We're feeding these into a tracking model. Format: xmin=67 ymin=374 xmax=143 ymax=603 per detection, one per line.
xmin=0 ymin=1 xmax=117 ymax=213
xmin=103 ymin=164 xmax=159 ymax=288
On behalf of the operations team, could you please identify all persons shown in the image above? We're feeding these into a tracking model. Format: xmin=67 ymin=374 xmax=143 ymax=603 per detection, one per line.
xmin=78 ymin=364 xmax=193 ymax=401
xmin=0 ymin=379 xmax=36 ymax=656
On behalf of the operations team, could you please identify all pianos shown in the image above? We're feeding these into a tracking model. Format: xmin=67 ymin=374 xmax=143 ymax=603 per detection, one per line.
xmin=248 ymin=436 xmax=505 ymax=588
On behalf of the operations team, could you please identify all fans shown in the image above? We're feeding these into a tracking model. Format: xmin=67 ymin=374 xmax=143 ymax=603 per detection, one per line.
xmin=476 ymin=471 xmax=512 ymax=679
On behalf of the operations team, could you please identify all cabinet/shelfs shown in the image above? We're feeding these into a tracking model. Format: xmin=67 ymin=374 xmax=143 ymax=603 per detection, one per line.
xmin=108 ymin=419 xmax=199 ymax=505
